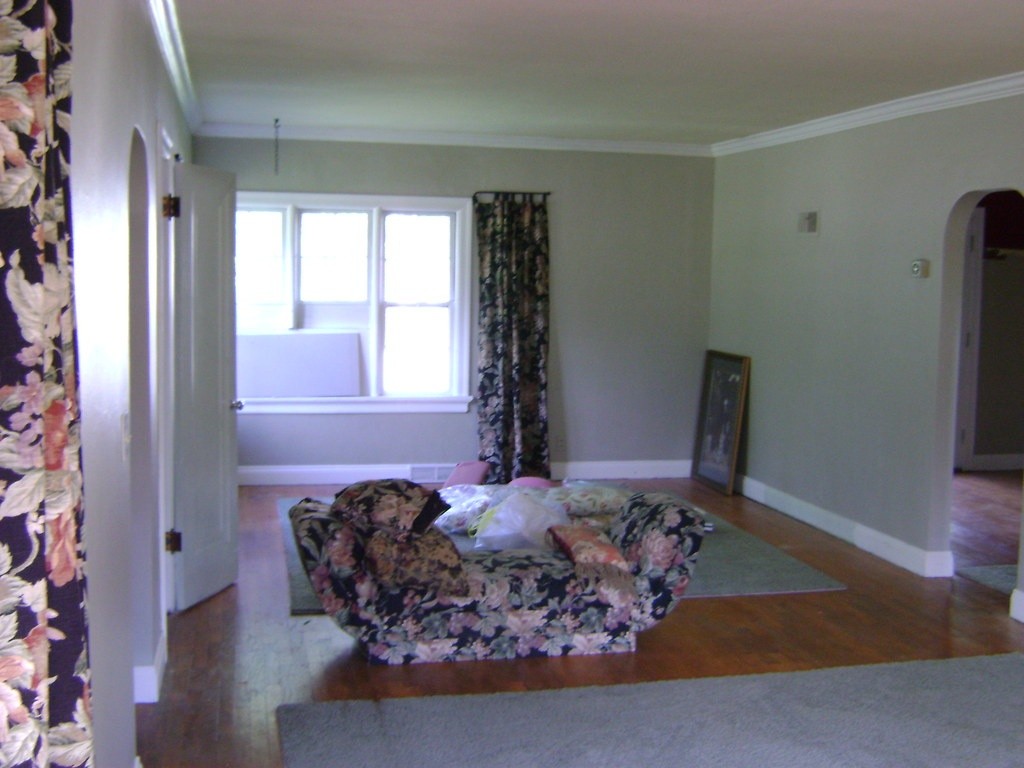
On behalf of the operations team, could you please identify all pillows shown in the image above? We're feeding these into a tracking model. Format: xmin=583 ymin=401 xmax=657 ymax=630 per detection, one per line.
xmin=552 ymin=488 xmax=625 ymax=513
xmin=508 ymin=477 xmax=554 ymax=488
xmin=365 ymin=526 xmax=469 ymax=594
xmin=332 ymin=478 xmax=431 ymax=530
xmin=549 ymin=525 xmax=629 ymax=571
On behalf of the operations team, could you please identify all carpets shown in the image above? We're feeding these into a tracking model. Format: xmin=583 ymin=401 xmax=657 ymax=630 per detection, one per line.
xmin=955 ymin=564 xmax=1017 ymax=596
xmin=277 ymin=650 xmax=1024 ymax=768
xmin=279 ymin=488 xmax=847 ymax=613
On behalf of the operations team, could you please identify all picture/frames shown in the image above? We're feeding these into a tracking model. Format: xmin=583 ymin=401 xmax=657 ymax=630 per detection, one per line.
xmin=691 ymin=349 xmax=751 ymax=497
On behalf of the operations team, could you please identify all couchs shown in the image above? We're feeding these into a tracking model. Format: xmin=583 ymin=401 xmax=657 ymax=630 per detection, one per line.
xmin=290 ymin=493 xmax=706 ymax=663
